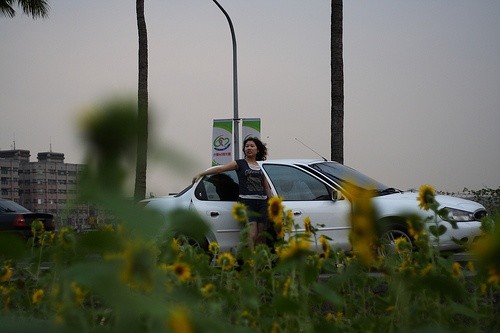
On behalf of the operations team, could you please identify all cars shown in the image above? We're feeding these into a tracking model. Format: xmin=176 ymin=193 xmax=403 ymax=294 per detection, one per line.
xmin=134 ymin=158 xmax=496 ymax=272
xmin=0 ymin=198 xmax=56 ymax=234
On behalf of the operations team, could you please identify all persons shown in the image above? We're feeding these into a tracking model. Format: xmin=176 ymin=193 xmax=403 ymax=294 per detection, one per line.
xmin=191 ymin=136 xmax=272 ymax=242
